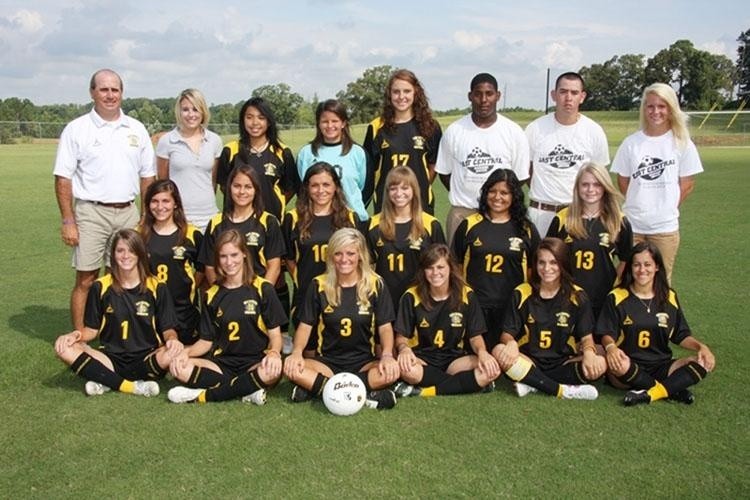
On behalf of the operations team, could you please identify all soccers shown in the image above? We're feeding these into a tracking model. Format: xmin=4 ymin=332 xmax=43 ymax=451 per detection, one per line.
xmin=323 ymin=373 xmax=367 ymax=416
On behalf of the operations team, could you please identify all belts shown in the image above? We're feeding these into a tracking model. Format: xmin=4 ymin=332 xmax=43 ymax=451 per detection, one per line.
xmin=87 ymin=199 xmax=135 ymax=208
xmin=529 ymin=199 xmax=570 ymax=215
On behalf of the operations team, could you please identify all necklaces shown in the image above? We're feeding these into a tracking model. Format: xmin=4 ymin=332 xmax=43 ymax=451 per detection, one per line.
xmin=638 ymin=299 xmax=653 ymax=313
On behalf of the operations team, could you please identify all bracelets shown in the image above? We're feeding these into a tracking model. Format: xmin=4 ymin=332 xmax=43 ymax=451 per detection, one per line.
xmin=605 ymin=342 xmax=615 ymax=351
xmin=62 ymin=218 xmax=75 ymax=224
xmin=73 ymin=330 xmax=82 ymax=343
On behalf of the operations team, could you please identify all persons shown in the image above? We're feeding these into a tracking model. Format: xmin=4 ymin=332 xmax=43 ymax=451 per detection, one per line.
xmin=284 ymin=227 xmax=401 ymax=408
xmin=52 ymin=69 xmax=157 ymax=330
xmin=53 ymin=228 xmax=185 ymax=398
xmin=281 ymin=163 xmax=363 ymax=328
xmin=135 ymin=180 xmax=206 ymax=344
xmin=453 ymin=168 xmax=543 ymax=341
xmin=524 ymin=72 xmax=612 ymax=239
xmin=357 ymin=165 xmax=445 ymax=319
xmin=164 ymin=229 xmax=290 ymax=405
xmin=297 ymin=99 xmax=371 ymax=229
xmin=218 ymin=96 xmax=296 ymax=224
xmin=156 ymin=88 xmax=224 ymax=236
xmin=491 ymin=236 xmax=607 ymax=400
xmin=594 ymin=242 xmax=716 ymax=406
xmin=609 ymin=83 xmax=706 ymax=288
xmin=545 ymin=162 xmax=631 ymax=320
xmin=434 ymin=73 xmax=530 ymax=246
xmin=393 ymin=243 xmax=501 ymax=397
xmin=361 ymin=70 xmax=450 ymax=217
xmin=196 ymin=163 xmax=290 ymax=327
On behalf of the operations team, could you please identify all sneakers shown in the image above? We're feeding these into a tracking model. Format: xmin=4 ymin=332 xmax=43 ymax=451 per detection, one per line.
xmin=670 ymin=388 xmax=694 ymax=405
xmin=85 ymin=379 xmax=112 ymax=396
xmin=511 ymin=381 xmax=539 ymax=398
xmin=622 ymin=389 xmax=651 ymax=406
xmin=241 ymin=389 xmax=267 ymax=406
xmin=132 ymin=379 xmax=160 ymax=398
xmin=289 ymin=385 xmax=313 ymax=402
xmin=282 ymin=334 xmax=294 ymax=354
xmin=393 ymin=382 xmax=415 ymax=399
xmin=166 ymin=385 xmax=205 ymax=404
xmin=364 ymin=389 xmax=397 ymax=410
xmin=561 ymin=384 xmax=599 ymax=401
xmin=480 ymin=380 xmax=495 ymax=395
